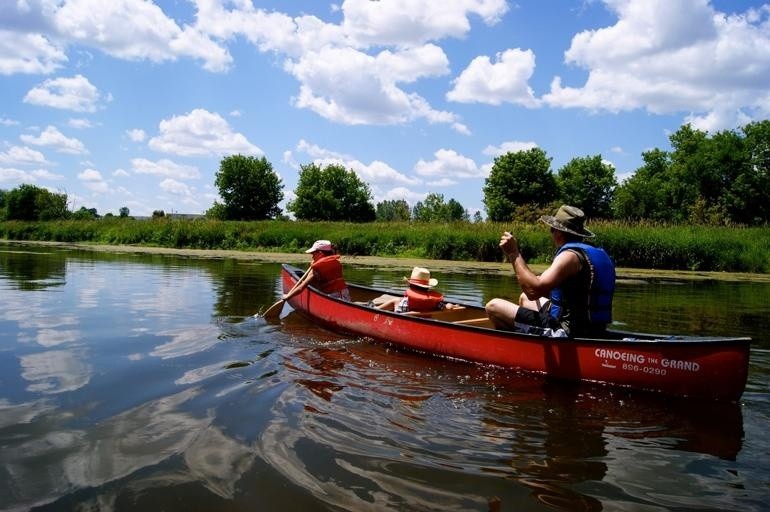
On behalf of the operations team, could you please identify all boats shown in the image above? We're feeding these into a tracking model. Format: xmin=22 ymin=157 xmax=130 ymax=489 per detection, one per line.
xmin=276 ymin=263 xmax=752 ymax=400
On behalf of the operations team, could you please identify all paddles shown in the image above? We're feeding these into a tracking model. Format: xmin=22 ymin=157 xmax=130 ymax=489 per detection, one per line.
xmin=262 ymin=267 xmax=313 ymax=318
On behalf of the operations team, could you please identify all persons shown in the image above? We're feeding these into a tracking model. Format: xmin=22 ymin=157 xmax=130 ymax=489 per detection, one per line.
xmin=280 ymin=238 xmax=354 ymax=305
xmin=362 ymin=267 xmax=460 ymax=313
xmin=484 ymin=204 xmax=617 ymax=339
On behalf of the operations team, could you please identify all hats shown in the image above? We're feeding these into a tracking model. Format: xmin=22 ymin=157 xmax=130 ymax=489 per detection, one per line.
xmin=305 ymin=238 xmax=333 ymax=253
xmin=539 ymin=205 xmax=595 ymax=239
xmin=402 ymin=266 xmax=438 ymax=289
